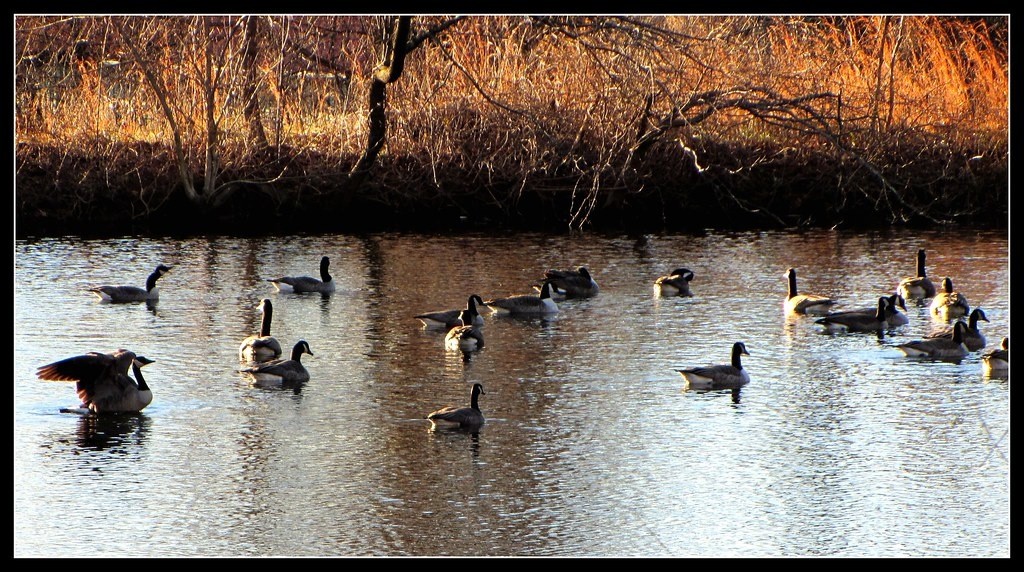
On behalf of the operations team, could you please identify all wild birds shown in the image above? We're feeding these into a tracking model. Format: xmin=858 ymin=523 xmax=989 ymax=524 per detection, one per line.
xmin=86 ymin=263 xmax=174 ymax=301
xmin=412 ymin=265 xmax=600 ymax=355
xmin=266 ymin=255 xmax=336 ymax=293
xmin=237 ymin=298 xmax=315 ymax=387
xmin=979 ymin=337 xmax=1008 ymax=370
xmin=422 ymin=383 xmax=486 ymax=432
xmin=782 ymin=248 xmax=991 ymax=358
xmin=674 ymin=342 xmax=751 ymax=390
xmin=35 ymin=348 xmax=156 ymax=413
xmin=653 ymin=268 xmax=694 ymax=297
